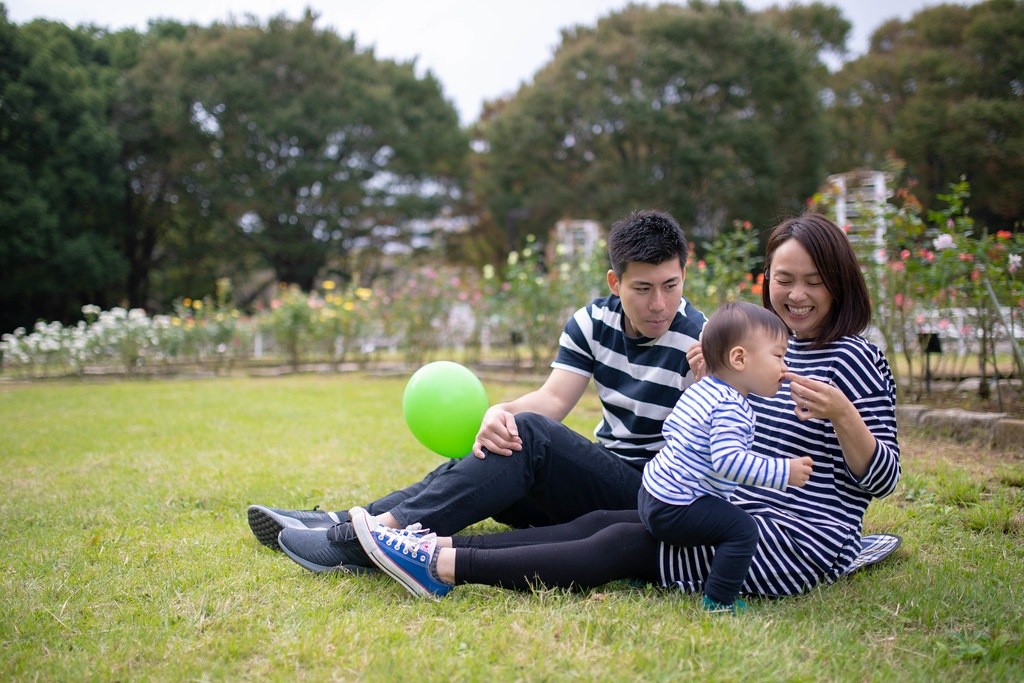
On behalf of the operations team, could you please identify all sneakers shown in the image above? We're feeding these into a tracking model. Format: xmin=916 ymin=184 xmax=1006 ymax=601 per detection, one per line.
xmin=248 ymin=504 xmax=339 ymax=551
xmin=348 ymin=506 xmax=455 ymax=601
xmin=277 ymin=518 xmax=379 ymax=575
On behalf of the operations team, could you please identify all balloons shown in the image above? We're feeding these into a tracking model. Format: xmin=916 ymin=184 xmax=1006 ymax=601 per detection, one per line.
xmin=403 ymin=360 xmax=487 ymax=458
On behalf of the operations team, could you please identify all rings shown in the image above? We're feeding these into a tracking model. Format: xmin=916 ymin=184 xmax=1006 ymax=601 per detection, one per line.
xmin=803 ymin=398 xmax=808 ymax=408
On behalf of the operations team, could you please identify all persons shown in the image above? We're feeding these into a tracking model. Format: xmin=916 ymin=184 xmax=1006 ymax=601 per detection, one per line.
xmin=638 ymin=301 xmax=813 ymax=613
xmin=350 ymin=213 xmax=901 ymax=606
xmin=247 ymin=207 xmax=710 ymax=581
